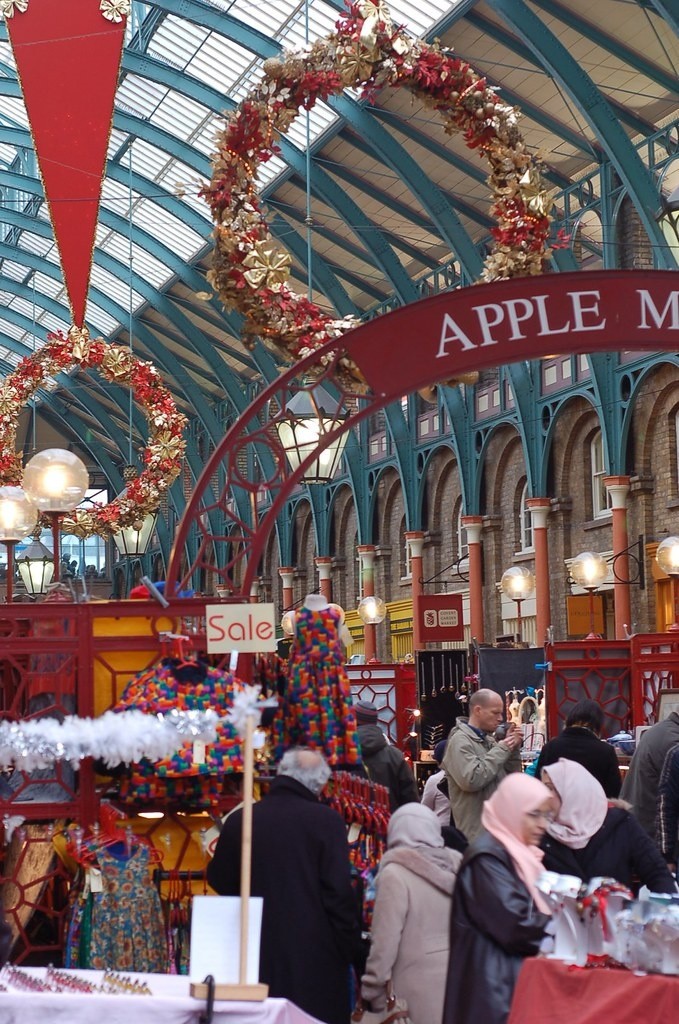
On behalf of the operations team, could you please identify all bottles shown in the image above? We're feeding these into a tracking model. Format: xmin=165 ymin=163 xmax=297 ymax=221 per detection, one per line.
xmin=579 ymin=877 xmax=679 ymax=975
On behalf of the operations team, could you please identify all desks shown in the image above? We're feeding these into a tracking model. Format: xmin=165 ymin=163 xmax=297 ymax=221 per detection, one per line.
xmin=499 ymin=955 xmax=679 ymax=1024
xmin=0 ymin=957 xmax=330 ymax=1024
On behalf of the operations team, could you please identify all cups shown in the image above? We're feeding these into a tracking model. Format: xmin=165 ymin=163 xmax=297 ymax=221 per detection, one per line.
xmin=614 ymin=747 xmax=627 ymax=759
xmin=618 ymin=739 xmax=636 ymax=756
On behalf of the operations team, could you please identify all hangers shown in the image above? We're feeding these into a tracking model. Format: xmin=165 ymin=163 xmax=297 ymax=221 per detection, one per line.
xmin=152 ymin=627 xmax=209 ymax=678
xmin=323 ymin=771 xmax=394 ymax=831
xmin=62 ymin=800 xmax=208 ymax=926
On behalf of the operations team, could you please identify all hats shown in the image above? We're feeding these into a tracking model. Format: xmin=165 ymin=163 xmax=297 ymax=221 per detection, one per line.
xmin=353 ymin=701 xmax=379 ymax=726
xmin=431 ymin=740 xmax=447 ymax=759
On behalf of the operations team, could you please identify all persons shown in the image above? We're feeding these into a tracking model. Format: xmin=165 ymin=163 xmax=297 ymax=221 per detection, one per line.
xmin=441 ymin=773 xmax=554 ymax=1024
xmin=534 ymin=699 xmax=622 ymax=799
xmin=439 ymin=689 xmax=524 ymax=847
xmin=618 ymin=712 xmax=679 ymax=835
xmin=361 ymin=802 xmax=463 ymax=1024
xmin=421 ymin=740 xmax=451 ymax=826
xmin=352 ymin=701 xmax=420 ymax=815
xmin=537 ymin=756 xmax=675 ymax=898
xmin=654 ymin=744 xmax=679 ymax=882
xmin=208 ymin=748 xmax=370 ymax=1024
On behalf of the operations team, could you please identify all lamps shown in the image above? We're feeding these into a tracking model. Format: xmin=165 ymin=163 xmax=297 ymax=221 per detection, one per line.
xmin=19 ymin=446 xmax=91 ymax=604
xmin=278 ymin=532 xmax=679 ymax=638
xmin=0 ymin=483 xmax=43 ymax=604
xmin=105 ymin=138 xmax=164 ymax=562
xmin=13 ymin=263 xmax=58 ymax=597
xmin=268 ymin=0 xmax=354 ymax=489
xmin=400 ymin=701 xmax=424 ymax=739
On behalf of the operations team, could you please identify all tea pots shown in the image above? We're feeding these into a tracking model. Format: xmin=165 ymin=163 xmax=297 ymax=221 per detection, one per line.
xmin=607 ymin=730 xmax=632 ymax=747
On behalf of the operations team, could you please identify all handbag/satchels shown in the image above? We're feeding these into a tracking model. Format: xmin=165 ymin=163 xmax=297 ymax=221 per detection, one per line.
xmin=349 ymin=979 xmax=413 ymax=1024
